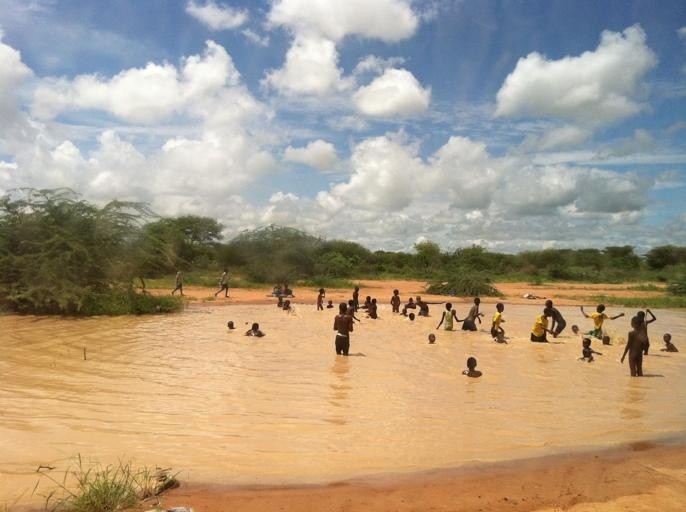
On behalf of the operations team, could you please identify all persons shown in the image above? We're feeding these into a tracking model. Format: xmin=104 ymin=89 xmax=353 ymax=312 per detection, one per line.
xmin=266 ymin=279 xmax=295 ymax=310
xmin=317 ymin=286 xmax=377 ymax=355
xmin=530 ymin=299 xmax=656 ymax=376
xmin=215 ymin=268 xmax=231 ymax=298
xmin=244 ymin=323 xmax=265 ymax=337
xmin=228 ymin=321 xmax=236 ymax=329
xmin=390 ymin=289 xmax=508 ymax=377
xmin=171 ymin=270 xmax=185 ymax=296
xmin=660 ymin=333 xmax=679 ymax=352
